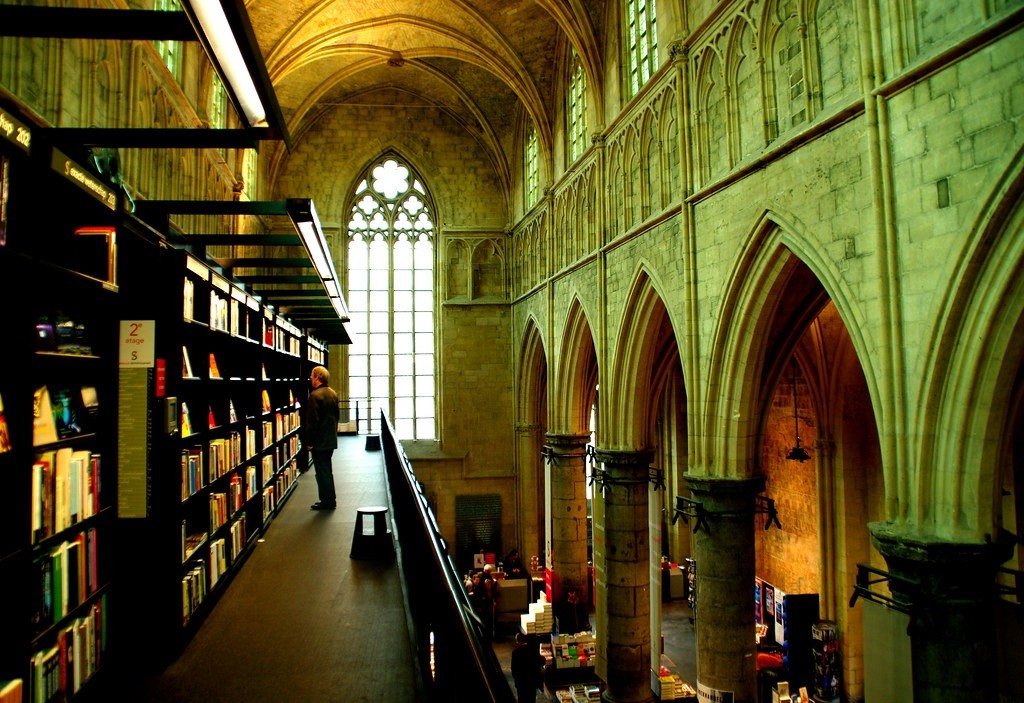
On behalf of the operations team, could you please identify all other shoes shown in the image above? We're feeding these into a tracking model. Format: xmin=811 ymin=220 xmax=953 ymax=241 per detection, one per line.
xmin=311 ymin=501 xmax=336 ymax=510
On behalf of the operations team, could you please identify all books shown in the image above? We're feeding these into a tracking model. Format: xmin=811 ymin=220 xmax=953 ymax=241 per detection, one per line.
xmin=539 ymin=643 xmax=552 ymax=657
xmin=181 ymin=276 xmax=324 ymax=624
xmin=778 ymin=681 xmax=809 ymax=703
xmin=0 ymin=158 xmax=109 ymax=703
xmin=659 ymin=666 xmax=696 ymax=700
xmin=556 ymin=684 xmax=601 ymax=703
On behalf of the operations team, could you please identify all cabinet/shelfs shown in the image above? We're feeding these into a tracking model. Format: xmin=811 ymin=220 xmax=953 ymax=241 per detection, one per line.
xmin=0 ymin=135 xmax=332 ymax=703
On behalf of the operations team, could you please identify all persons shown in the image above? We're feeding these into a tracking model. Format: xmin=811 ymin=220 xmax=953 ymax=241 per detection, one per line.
xmin=511 ymin=633 xmax=552 ymax=703
xmin=661 ymin=557 xmax=671 ymax=602
xmin=301 ymin=366 xmax=340 ymax=509
xmin=465 ymin=564 xmax=497 ymax=637
xmin=506 ymin=549 xmax=522 ymax=580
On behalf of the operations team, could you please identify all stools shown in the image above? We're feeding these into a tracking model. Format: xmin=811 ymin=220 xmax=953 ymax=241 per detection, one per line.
xmin=365 ymin=436 xmax=381 ymax=452
xmin=349 ymin=506 xmax=395 ymax=562
